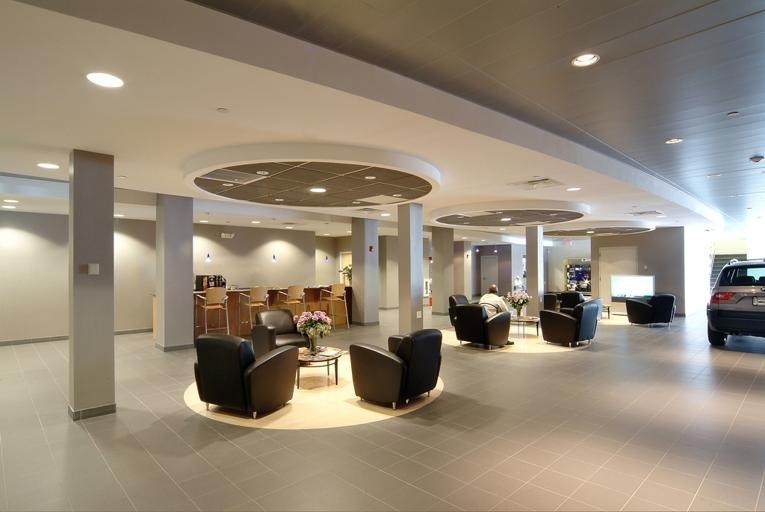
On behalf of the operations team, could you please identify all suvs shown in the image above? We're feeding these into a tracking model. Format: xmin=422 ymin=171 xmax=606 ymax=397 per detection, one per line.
xmin=707 ymin=258 xmax=765 ymax=347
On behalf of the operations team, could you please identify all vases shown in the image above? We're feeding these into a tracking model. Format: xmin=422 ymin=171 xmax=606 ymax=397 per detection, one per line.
xmin=516 ymin=305 xmax=524 ymax=317
xmin=308 ymin=334 xmax=320 ymax=357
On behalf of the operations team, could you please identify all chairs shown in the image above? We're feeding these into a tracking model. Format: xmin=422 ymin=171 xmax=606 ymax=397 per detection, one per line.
xmin=236 ymin=285 xmax=271 ymax=337
xmin=540 ymin=288 xmax=679 ymax=350
xmin=346 ymin=326 xmax=442 ymax=410
xmin=448 ymin=294 xmax=480 ymax=327
xmin=193 ymin=286 xmax=230 ymax=346
xmin=191 ymin=331 xmax=300 ymax=421
xmin=275 ymin=284 xmax=307 ymax=316
xmin=452 ymin=303 xmax=511 ymax=350
xmin=249 ymin=308 xmax=309 ymax=357
xmin=318 ymin=282 xmax=350 ymax=332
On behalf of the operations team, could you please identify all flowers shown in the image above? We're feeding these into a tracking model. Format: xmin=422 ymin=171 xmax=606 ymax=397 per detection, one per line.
xmin=506 ymin=288 xmax=534 ymax=318
xmin=291 ymin=308 xmax=333 ymax=354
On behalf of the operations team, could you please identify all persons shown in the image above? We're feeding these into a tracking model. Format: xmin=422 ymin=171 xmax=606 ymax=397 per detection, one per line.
xmin=478 ymin=284 xmax=518 ymax=346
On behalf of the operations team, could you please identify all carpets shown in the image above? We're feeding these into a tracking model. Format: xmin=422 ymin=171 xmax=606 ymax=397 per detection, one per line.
xmin=596 ymin=309 xmax=646 ymax=327
xmin=182 ymin=349 xmax=447 ymax=431
xmin=437 ymin=324 xmax=593 ymax=353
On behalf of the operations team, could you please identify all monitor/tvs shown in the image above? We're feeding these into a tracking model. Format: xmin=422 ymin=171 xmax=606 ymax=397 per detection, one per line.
xmin=610 ymin=274 xmax=656 ymax=302
